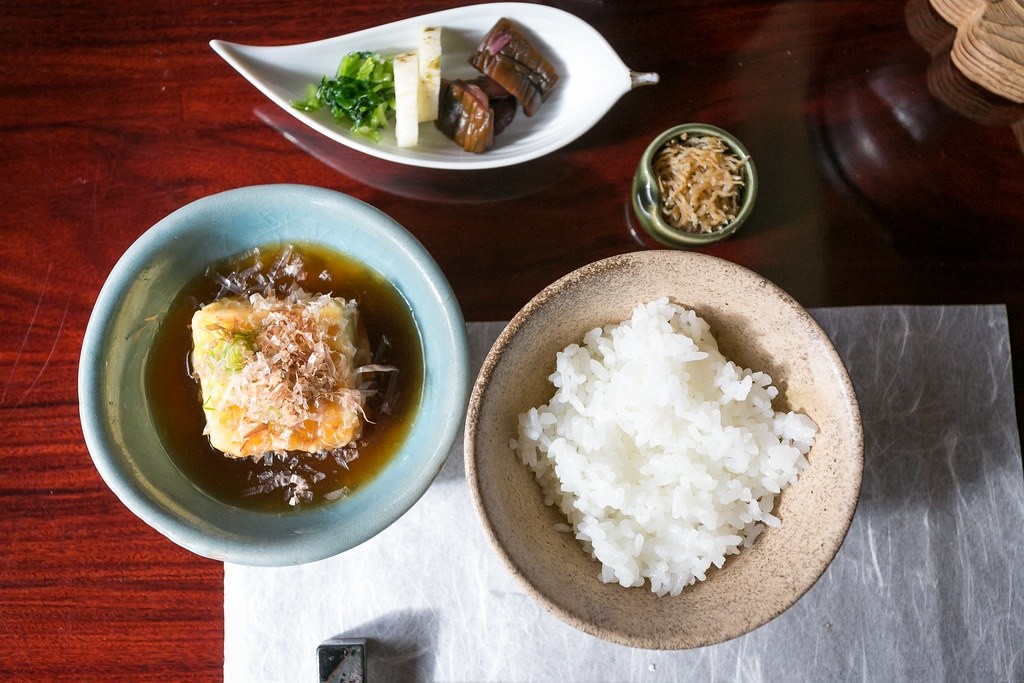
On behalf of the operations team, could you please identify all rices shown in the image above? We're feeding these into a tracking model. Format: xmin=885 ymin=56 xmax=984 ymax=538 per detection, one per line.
xmin=508 ymin=296 xmax=819 ymax=598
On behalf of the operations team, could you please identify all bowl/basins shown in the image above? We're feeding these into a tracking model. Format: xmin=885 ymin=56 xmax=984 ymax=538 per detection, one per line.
xmin=77 ymin=184 xmax=471 ymax=566
xmin=464 ymin=250 xmax=865 ymax=650
xmin=632 ymin=123 xmax=759 ymax=248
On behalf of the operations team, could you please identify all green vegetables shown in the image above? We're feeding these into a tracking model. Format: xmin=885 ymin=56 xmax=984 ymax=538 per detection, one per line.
xmin=288 ymin=50 xmax=396 ymax=144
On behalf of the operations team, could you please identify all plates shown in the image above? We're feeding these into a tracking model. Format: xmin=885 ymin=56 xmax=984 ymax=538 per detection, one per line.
xmin=208 ymin=2 xmax=659 ymax=169
